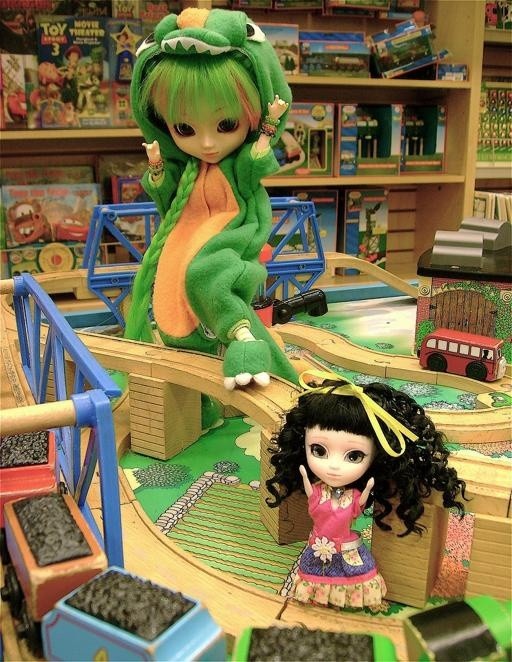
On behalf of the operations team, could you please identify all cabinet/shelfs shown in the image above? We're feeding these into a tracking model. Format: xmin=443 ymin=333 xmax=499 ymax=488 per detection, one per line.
xmin=0 ymin=0 xmax=512 ymax=302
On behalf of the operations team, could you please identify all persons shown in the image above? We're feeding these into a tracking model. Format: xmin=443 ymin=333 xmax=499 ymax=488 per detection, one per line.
xmin=130 ymin=8 xmax=303 ymax=433
xmin=0 ymin=0 xmax=511 ymax=661
xmin=265 ymin=369 xmax=472 ymax=609
